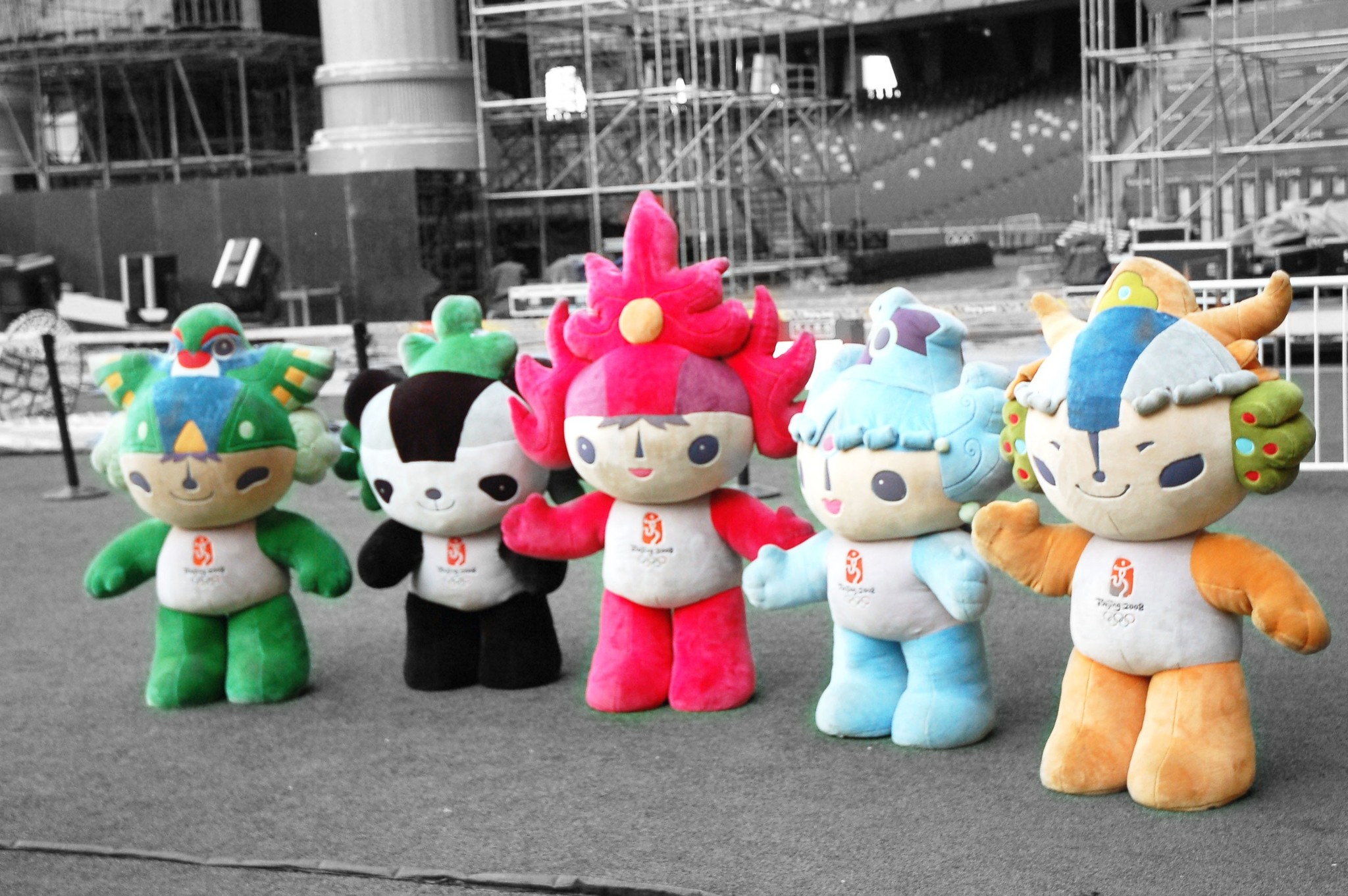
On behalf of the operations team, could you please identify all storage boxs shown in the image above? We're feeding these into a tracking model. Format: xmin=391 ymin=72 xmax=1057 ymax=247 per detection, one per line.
xmin=1252 ymin=244 xmax=1326 ymax=293
xmin=1128 ymin=242 xmax=1254 ymax=302
xmin=1131 ymin=225 xmax=1198 ymax=244
xmin=1317 ymin=240 xmax=1348 ymax=277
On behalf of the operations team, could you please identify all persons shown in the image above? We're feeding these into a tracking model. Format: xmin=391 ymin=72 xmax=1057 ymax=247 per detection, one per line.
xmin=489 ymin=251 xmax=528 ymax=319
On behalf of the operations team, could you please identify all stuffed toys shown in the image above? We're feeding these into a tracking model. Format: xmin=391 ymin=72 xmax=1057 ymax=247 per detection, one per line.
xmin=971 ymin=255 xmax=1331 ymax=813
xmin=501 ymin=188 xmax=816 ymax=712
xmin=331 ymin=294 xmax=587 ymax=692
xmin=741 ymin=287 xmax=1010 ymax=750
xmin=81 ymin=301 xmax=353 ymax=708
